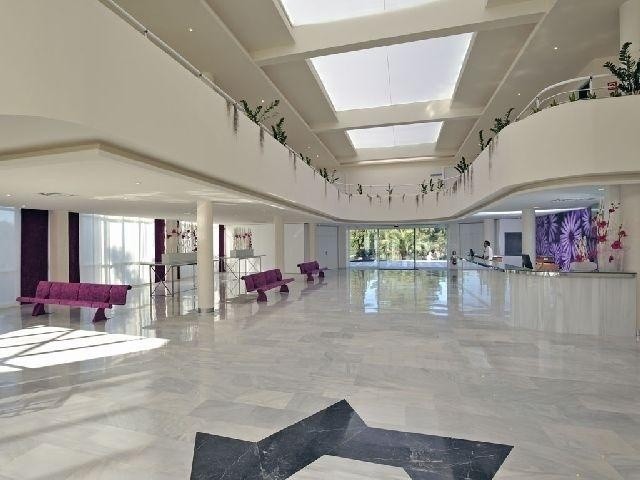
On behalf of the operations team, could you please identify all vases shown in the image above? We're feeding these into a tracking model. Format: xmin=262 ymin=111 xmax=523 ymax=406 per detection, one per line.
xmin=597 ymin=244 xmax=624 ymax=272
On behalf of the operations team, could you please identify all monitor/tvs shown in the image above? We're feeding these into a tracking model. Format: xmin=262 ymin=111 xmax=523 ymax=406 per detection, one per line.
xmin=521 ymin=254 xmax=533 ymax=269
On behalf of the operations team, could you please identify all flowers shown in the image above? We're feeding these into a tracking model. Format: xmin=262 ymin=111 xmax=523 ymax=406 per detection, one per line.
xmin=591 ymin=200 xmax=631 ymax=250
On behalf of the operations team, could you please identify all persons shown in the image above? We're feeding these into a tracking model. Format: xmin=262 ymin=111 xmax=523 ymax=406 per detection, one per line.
xmin=481 ymin=239 xmax=493 ymax=268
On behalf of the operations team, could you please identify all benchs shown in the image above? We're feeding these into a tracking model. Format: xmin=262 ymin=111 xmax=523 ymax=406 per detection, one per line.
xmin=240 ymin=261 xmax=328 ymax=302
xmin=16 ymin=280 xmax=132 ymax=322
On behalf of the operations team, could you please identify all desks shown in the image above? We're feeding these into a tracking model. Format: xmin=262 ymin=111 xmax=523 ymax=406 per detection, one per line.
xmin=139 ymin=255 xmax=267 ymax=298
